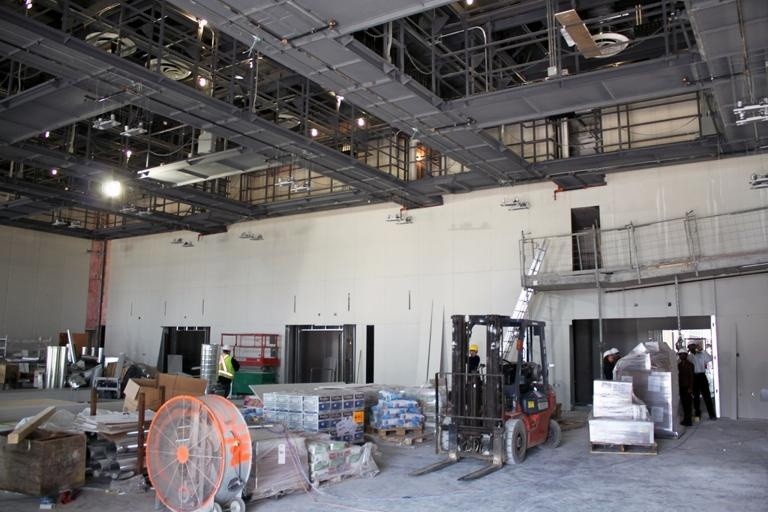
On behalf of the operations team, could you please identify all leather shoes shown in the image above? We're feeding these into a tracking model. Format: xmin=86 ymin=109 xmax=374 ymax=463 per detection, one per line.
xmin=709 ymin=414 xmax=716 ymax=420
xmin=679 ymin=420 xmax=694 ymax=426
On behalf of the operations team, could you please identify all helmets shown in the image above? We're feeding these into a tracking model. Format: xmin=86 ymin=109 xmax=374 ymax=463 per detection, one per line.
xmin=611 ymin=347 xmax=620 ymax=354
xmin=469 ymin=344 xmax=478 ymax=351
xmin=222 ymin=344 xmax=231 ymax=351
xmin=602 ymin=350 xmax=613 ymax=360
xmin=677 ymin=348 xmax=689 ymax=355
xmin=687 ymin=340 xmax=697 ymax=346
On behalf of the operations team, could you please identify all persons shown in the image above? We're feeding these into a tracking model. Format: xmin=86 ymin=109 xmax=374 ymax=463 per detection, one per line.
xmin=601 ymin=349 xmax=616 ymax=381
xmin=217 ymin=344 xmax=240 ymax=398
xmin=610 ymin=347 xmax=621 ymax=363
xmin=674 ymin=337 xmax=717 ymax=427
xmin=465 ymin=343 xmax=480 ymax=383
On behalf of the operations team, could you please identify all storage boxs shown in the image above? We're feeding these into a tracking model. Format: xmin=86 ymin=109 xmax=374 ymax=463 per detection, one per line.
xmin=366 ymin=382 xmax=445 ymax=430
xmin=0 ymin=427 xmax=87 ymax=498
xmin=262 ymin=391 xmax=365 ymax=444
xmin=122 ymin=370 xmax=209 ymax=412
xmin=0 ymin=363 xmax=20 ymax=384
xmin=586 ymin=366 xmax=680 ymax=447
xmin=306 ymin=438 xmax=365 ymax=479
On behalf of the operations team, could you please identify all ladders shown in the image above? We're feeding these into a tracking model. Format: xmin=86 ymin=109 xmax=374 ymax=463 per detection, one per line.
xmin=499 ymin=237 xmax=551 ymax=364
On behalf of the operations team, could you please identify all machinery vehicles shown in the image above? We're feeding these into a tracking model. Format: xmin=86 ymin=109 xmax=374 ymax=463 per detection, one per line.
xmin=406 ymin=312 xmax=563 ymax=482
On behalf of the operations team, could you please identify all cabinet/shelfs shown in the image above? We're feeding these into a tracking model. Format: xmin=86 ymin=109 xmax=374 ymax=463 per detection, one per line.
xmin=59 ymin=332 xmax=89 ymax=359
